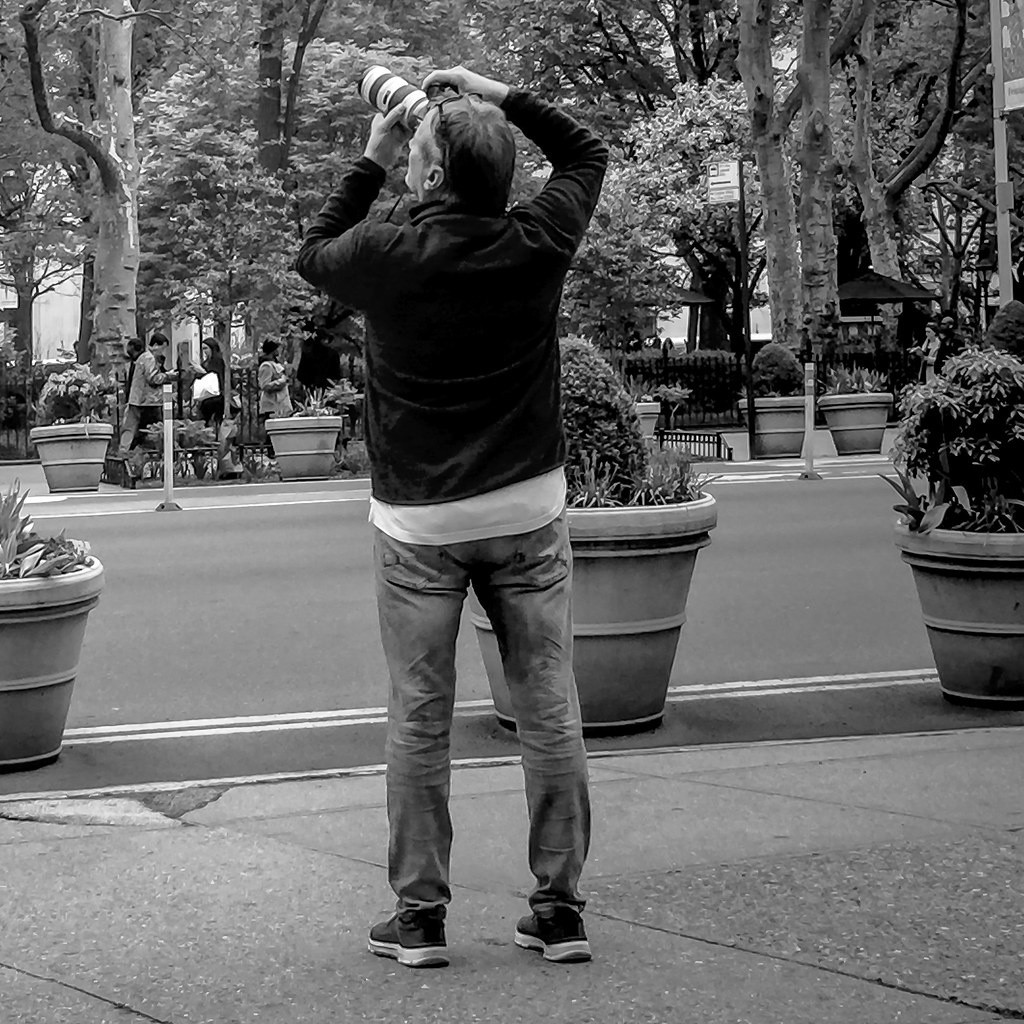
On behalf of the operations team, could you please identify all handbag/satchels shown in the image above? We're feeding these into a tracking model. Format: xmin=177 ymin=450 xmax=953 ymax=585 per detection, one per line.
xmin=193 ymin=372 xmax=220 ymax=401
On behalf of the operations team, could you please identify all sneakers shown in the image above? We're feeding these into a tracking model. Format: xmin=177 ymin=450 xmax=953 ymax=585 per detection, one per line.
xmin=516 ymin=906 xmax=592 ymax=962
xmin=368 ymin=912 xmax=449 ymax=966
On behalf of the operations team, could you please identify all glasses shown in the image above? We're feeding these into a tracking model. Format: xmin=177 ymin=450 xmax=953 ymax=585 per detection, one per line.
xmin=433 ymin=93 xmax=483 ymax=167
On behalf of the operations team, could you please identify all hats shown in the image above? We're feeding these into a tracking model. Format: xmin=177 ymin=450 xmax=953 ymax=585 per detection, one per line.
xmin=262 ymin=338 xmax=281 ymax=353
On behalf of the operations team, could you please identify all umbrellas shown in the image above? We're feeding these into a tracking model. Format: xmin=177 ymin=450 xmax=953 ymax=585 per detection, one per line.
xmin=836 ymin=271 xmax=943 ymax=337
xmin=637 ymin=285 xmax=716 ymax=305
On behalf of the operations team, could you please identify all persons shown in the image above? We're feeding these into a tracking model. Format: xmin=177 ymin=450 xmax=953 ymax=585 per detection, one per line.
xmin=117 ymin=331 xmax=179 ymax=457
xmin=297 ymin=325 xmax=340 ymax=414
xmin=293 ymin=61 xmax=613 ymax=970
xmin=935 ymin=316 xmax=965 ymax=378
xmin=256 ymin=335 xmax=294 ymax=461
xmin=187 ymin=336 xmax=224 ymax=436
xmin=125 ymin=336 xmax=147 ymax=423
xmin=911 ymin=322 xmax=941 ymax=385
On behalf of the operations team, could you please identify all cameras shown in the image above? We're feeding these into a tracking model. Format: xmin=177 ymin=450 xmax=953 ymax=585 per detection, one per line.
xmin=357 ymin=66 xmax=460 ymax=134
